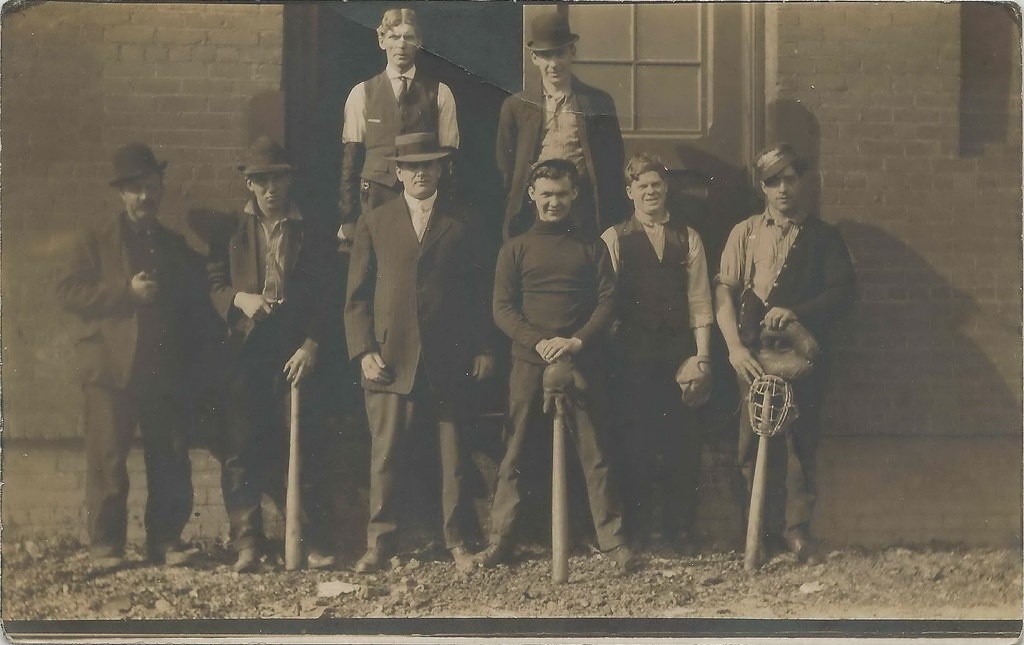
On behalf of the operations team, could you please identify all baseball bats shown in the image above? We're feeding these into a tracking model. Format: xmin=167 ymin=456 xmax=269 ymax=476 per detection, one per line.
xmin=286 ymin=377 xmax=304 ymax=571
xmin=545 ymin=394 xmax=577 ymax=583
xmin=745 ymin=391 xmax=773 ymax=571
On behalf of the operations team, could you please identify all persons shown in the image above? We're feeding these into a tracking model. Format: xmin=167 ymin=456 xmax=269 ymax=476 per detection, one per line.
xmin=599 ymin=154 xmax=714 ymax=551
xmin=464 ymin=158 xmax=641 ymax=571
xmin=59 ymin=142 xmax=207 ymax=570
xmin=344 ymin=133 xmax=499 ymax=574
xmin=337 ymin=9 xmax=460 ymax=251
xmin=496 ymin=14 xmax=625 ymax=244
xmin=716 ymin=145 xmax=858 ymax=560
xmin=207 ymin=137 xmax=341 ymax=573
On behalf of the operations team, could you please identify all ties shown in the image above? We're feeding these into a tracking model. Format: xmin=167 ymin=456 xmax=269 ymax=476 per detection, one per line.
xmin=399 ymin=77 xmax=409 ymax=108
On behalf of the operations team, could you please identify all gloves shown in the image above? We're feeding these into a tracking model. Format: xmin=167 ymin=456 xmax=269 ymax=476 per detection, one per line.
xmin=676 ymin=356 xmax=714 ymax=407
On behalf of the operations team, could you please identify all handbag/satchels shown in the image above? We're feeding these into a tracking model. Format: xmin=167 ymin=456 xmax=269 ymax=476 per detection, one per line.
xmin=732 ymin=287 xmax=766 ymax=343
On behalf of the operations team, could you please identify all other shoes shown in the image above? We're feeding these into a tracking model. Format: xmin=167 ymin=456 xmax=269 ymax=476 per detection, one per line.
xmin=146 ymin=544 xmax=187 ymax=565
xmin=673 ymin=528 xmax=698 ymax=559
xmin=95 ymin=555 xmax=122 ymax=569
xmin=627 ymin=532 xmax=648 ymax=553
xmin=471 ymin=542 xmax=513 ymax=564
xmin=234 ymin=547 xmax=260 ymax=571
xmin=302 ymin=547 xmax=335 ymax=568
xmin=787 ymin=537 xmax=820 ymax=565
xmin=604 ymin=545 xmax=635 ymax=569
xmin=450 ymin=545 xmax=477 ymax=569
xmin=355 ymin=549 xmax=384 ymax=571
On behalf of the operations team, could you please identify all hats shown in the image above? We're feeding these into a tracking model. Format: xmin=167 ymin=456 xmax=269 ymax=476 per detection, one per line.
xmin=108 ymin=141 xmax=170 ymax=187
xmin=383 ymin=133 xmax=455 ymax=162
xmin=751 ymin=142 xmax=798 ymax=183
xmin=526 ymin=13 xmax=579 ymax=52
xmin=236 ymin=137 xmax=292 ymax=172
